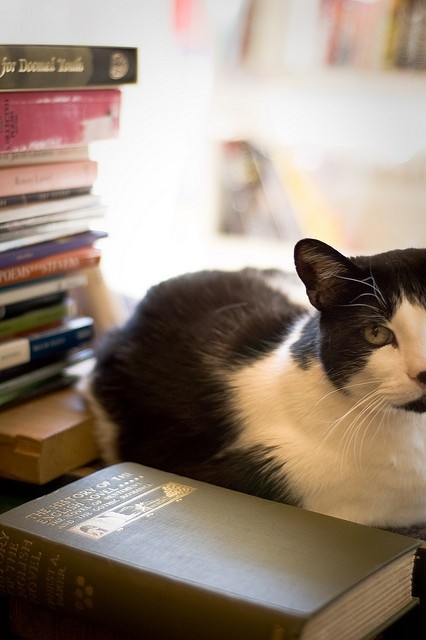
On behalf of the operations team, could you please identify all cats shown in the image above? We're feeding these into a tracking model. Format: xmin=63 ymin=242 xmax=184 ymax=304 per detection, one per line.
xmin=82 ymin=239 xmax=426 ymax=530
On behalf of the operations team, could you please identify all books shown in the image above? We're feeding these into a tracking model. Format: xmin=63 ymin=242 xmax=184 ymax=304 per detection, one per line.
xmin=0 ymin=461 xmax=426 ymax=640
